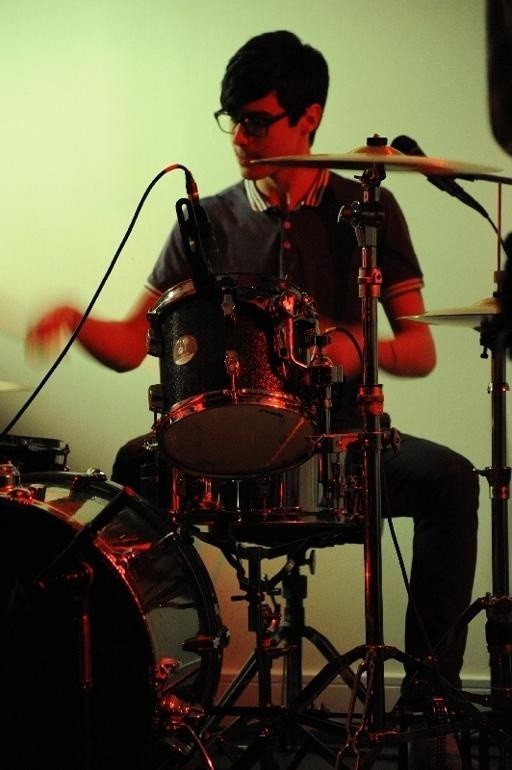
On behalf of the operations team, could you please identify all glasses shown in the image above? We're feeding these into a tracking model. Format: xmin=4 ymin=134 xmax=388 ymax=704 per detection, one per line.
xmin=214 ymin=108 xmax=289 ymax=137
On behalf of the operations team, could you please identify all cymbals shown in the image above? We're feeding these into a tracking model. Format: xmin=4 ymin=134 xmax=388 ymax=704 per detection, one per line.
xmin=248 ymin=151 xmax=512 ymax=183
xmin=3 ymin=381 xmax=27 ymax=394
xmin=392 ymin=295 xmax=502 ymax=328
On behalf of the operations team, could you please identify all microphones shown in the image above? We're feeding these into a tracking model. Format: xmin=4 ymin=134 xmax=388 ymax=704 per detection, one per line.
xmin=388 ymin=131 xmax=489 ymax=220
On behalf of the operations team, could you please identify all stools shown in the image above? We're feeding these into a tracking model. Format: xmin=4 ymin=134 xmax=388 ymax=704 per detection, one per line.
xmin=187 ymin=517 xmax=368 ymax=756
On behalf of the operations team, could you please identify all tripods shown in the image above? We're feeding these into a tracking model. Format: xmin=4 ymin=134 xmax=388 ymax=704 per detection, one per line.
xmin=180 ymin=200 xmax=512 ymax=770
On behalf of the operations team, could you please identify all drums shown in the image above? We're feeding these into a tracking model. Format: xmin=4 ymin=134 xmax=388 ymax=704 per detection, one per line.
xmin=0 ymin=466 xmax=228 ymax=770
xmin=240 ymin=424 xmax=372 ymax=549
xmin=171 ymin=469 xmax=235 ymax=525
xmin=143 ymin=271 xmax=322 ymax=481
xmin=0 ymin=432 xmax=69 ymax=478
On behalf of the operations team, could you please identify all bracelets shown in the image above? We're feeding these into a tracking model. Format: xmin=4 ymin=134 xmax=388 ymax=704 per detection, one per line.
xmin=384 ymin=338 xmax=397 ymax=374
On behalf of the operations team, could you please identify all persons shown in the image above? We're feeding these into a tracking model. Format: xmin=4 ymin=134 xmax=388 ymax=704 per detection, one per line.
xmin=21 ymin=29 xmax=484 ymax=733
